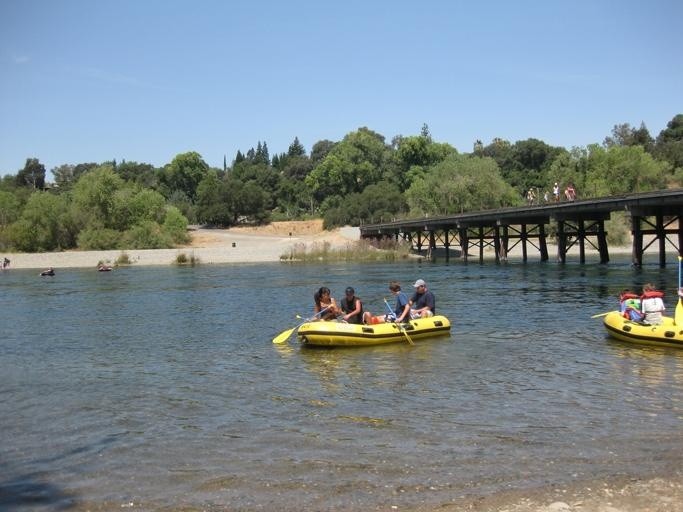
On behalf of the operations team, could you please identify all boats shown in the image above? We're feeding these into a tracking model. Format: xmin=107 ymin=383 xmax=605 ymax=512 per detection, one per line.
xmin=43 ymin=269 xmax=55 ymax=276
xmin=298 ymin=314 xmax=450 ymax=347
xmin=604 ymin=310 xmax=682 ymax=349
xmin=99 ymin=265 xmax=111 ymax=270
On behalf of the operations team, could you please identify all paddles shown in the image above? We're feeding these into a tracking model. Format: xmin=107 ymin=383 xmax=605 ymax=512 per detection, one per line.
xmin=674 ymin=256 xmax=683 ymax=328
xmin=383 ymin=298 xmax=415 ymax=346
xmin=272 ymin=304 xmax=334 ymax=344
xmin=591 ymin=310 xmax=622 ymax=318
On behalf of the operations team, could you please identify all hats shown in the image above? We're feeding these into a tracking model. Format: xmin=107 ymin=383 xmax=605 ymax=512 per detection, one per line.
xmin=346 ymin=287 xmax=354 ymax=293
xmin=413 ymin=280 xmax=425 ymax=288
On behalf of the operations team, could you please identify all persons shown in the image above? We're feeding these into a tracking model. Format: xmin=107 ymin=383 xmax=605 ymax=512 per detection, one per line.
xmin=676 ymin=287 xmax=683 ymax=297
xmin=338 ymin=287 xmax=365 ymax=323
xmin=625 ymin=282 xmax=666 ymax=325
xmin=3 ymin=258 xmax=10 ymax=268
xmin=44 ymin=266 xmax=53 ymax=273
xmin=310 ymin=286 xmax=341 ymax=321
xmin=409 ymin=279 xmax=435 ymax=320
xmin=526 ymin=181 xmax=576 ymax=208
xmin=620 ymin=288 xmax=645 ymax=324
xmin=389 ymin=281 xmax=410 ymax=322
